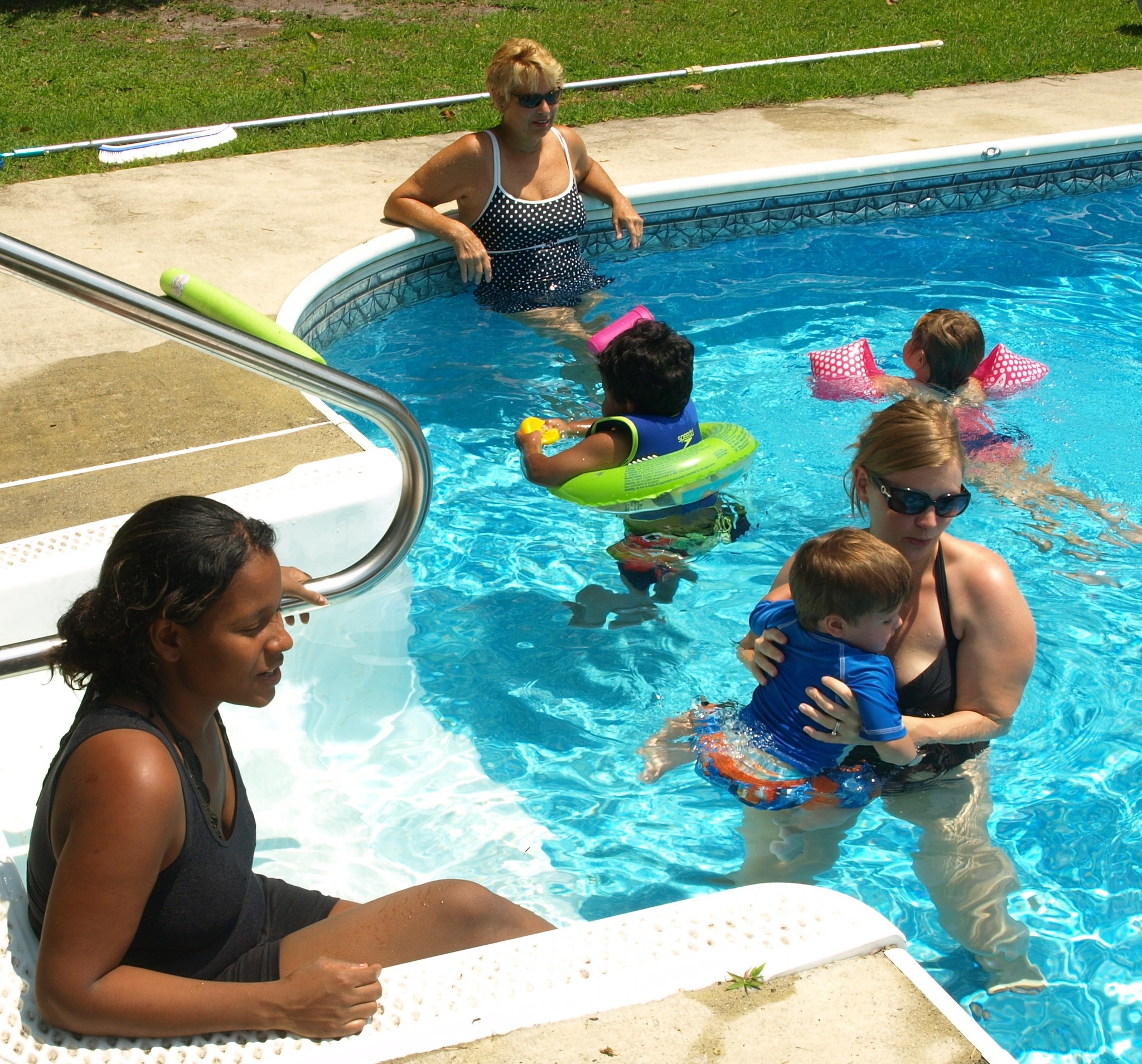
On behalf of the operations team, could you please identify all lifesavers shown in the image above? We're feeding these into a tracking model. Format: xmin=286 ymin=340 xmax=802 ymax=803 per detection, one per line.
xmin=159 ymin=268 xmax=326 ymax=367
xmin=547 ymin=423 xmax=757 ymax=516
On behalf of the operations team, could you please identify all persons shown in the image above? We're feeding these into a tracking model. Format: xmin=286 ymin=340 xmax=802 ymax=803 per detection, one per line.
xmin=749 ymin=396 xmax=1036 ymax=744
xmin=529 ymin=316 xmax=718 ymax=520
xmin=383 ymin=38 xmax=643 ymax=305
xmin=27 ymin=495 xmax=557 ymax=1038
xmin=902 ymin=308 xmax=985 ymax=385
xmin=749 ymin=528 xmax=915 ymax=760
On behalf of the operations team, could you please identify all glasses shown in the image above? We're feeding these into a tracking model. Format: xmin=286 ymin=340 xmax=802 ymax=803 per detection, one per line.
xmin=873 ymin=477 xmax=971 ymax=518
xmin=511 ymin=87 xmax=562 ymax=108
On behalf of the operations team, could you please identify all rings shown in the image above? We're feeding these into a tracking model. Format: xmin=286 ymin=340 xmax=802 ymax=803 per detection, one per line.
xmin=832 ymin=721 xmax=840 ymax=736
